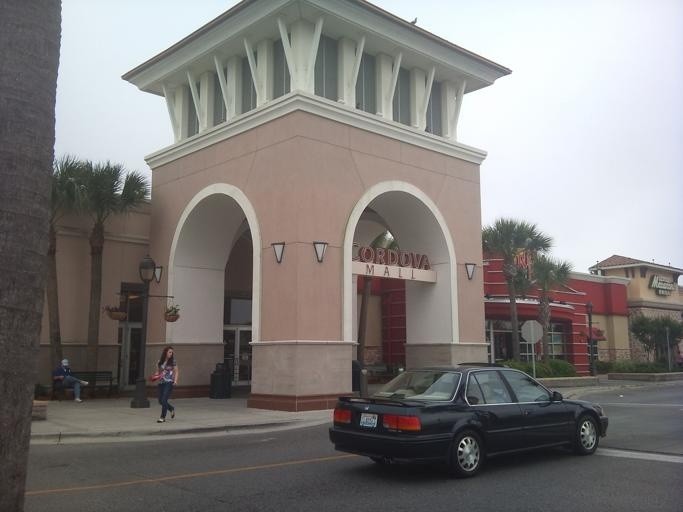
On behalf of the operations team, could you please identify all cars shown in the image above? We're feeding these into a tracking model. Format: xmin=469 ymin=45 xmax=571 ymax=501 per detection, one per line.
xmin=329 ymin=362 xmax=609 ymax=479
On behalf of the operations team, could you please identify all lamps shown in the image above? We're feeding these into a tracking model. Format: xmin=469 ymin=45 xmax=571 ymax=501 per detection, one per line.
xmin=270 ymin=242 xmax=286 ymax=263
xmin=313 ymin=240 xmax=328 ymax=263
xmin=154 ymin=266 xmax=163 ymax=283
xmin=465 ymin=262 xmax=476 ymax=280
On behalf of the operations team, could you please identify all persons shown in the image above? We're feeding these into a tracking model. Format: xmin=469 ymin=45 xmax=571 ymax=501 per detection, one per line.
xmin=153 ymin=346 xmax=179 ymax=423
xmin=51 ymin=359 xmax=89 ymax=403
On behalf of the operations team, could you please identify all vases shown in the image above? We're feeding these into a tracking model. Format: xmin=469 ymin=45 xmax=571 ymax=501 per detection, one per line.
xmin=108 ymin=312 xmax=127 ymax=320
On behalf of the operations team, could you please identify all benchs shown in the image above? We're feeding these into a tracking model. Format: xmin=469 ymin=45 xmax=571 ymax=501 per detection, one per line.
xmin=50 ymin=370 xmax=121 ymax=400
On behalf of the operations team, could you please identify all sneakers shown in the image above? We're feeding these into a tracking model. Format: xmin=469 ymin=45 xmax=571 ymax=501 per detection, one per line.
xmin=80 ymin=381 xmax=88 ymax=387
xmin=157 ymin=417 xmax=165 ymax=422
xmin=74 ymin=398 xmax=82 ymax=403
xmin=171 ymin=408 xmax=175 ymax=418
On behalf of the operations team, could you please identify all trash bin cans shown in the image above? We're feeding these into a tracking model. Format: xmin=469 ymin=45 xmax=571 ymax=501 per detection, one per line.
xmin=210 ymin=363 xmax=232 ymax=399
xmin=352 ymin=360 xmax=361 ymax=391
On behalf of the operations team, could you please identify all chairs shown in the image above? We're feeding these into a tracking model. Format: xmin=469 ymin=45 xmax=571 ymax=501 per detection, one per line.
xmin=467 ymin=383 xmax=495 ymax=404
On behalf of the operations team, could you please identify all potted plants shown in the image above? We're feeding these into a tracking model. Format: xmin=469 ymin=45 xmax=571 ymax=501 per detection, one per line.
xmin=163 ymin=304 xmax=181 ymax=322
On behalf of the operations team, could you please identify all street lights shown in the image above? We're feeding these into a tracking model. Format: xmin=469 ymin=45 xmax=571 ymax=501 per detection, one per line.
xmin=584 ymin=301 xmax=597 ymax=376
xmin=131 ymin=254 xmax=155 ymax=408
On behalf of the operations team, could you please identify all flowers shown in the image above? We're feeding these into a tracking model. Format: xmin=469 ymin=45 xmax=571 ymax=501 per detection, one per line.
xmin=103 ymin=304 xmax=127 ymax=312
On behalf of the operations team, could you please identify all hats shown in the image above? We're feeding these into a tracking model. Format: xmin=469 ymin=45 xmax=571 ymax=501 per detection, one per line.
xmin=61 ymin=359 xmax=68 ymax=366
